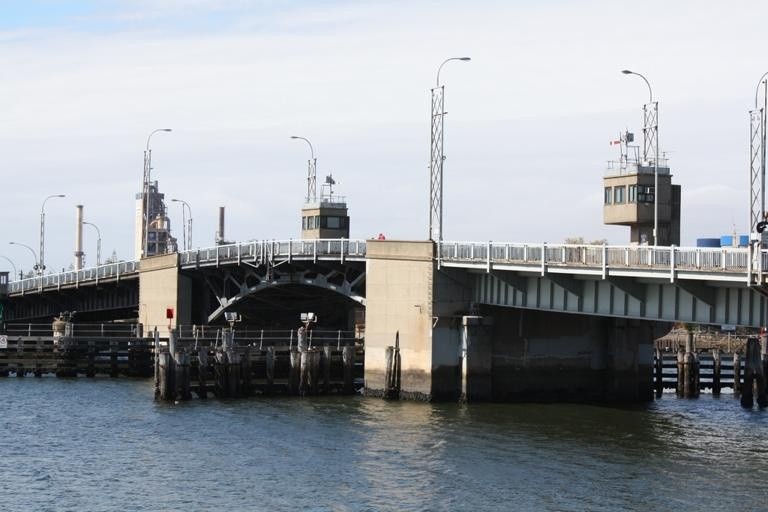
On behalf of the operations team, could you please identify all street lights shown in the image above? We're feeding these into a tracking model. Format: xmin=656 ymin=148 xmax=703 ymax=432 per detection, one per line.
xmin=623 ymin=69 xmax=659 ymax=164
xmin=430 ymin=56 xmax=470 ymax=240
xmin=142 ymin=128 xmax=172 ymax=191
xmin=82 ymin=221 xmax=102 ymax=267
xmin=171 ymin=198 xmax=193 ymax=251
xmin=9 ymin=243 xmax=37 ymax=274
xmin=291 ymin=136 xmax=317 ymax=204
xmin=39 ymin=195 xmax=65 ymax=274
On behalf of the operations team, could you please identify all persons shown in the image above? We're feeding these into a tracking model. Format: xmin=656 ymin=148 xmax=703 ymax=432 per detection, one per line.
xmin=378 ymin=233 xmax=385 ymax=240
